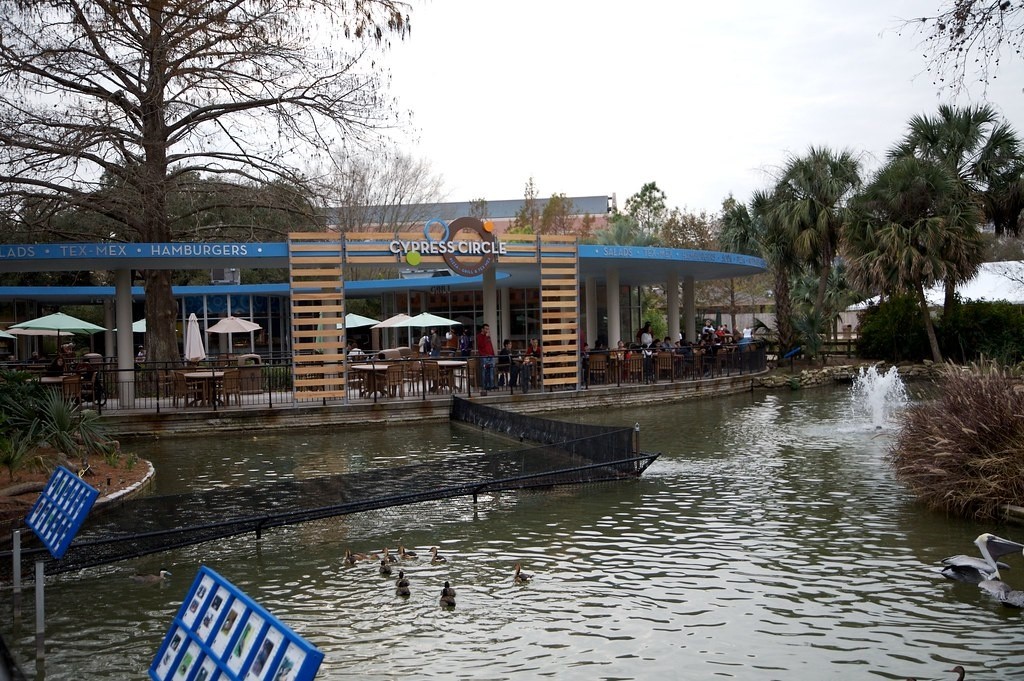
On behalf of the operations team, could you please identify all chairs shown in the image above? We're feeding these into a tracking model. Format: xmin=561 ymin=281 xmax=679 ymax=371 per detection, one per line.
xmin=345 ymin=343 xmax=738 ymax=399
xmin=158 ymin=365 xmax=243 ymax=409
xmin=61 ymin=368 xmax=107 ymax=413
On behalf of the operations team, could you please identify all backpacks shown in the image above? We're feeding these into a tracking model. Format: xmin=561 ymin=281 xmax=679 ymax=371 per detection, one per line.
xmin=423 ymin=336 xmax=431 ymax=352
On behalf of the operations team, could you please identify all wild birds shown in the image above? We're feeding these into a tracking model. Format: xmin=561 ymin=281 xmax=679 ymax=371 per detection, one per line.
xmin=942 ymin=666 xmax=967 ymax=681
xmin=429 ymin=547 xmax=446 ymax=564
xmin=346 ymin=546 xmax=418 ymax=596
xmin=937 ymin=533 xmax=1024 ymax=582
xmin=440 ymin=581 xmax=456 ymax=609
xmin=513 ymin=563 xmax=532 ymax=586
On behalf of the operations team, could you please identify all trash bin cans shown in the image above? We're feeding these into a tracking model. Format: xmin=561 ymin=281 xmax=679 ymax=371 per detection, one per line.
xmin=81 ymin=353 xmax=104 ymax=373
xmin=842 ymin=323 xmax=852 ymax=340
xmin=236 ymin=353 xmax=263 ymax=394
xmin=377 ymin=349 xmax=401 ymax=360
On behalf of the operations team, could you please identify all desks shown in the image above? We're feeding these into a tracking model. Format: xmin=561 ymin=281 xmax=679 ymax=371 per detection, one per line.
xmin=184 ymin=372 xmax=225 ymax=408
xmin=351 ymin=365 xmax=393 ymax=400
xmin=419 ymin=361 xmax=467 ymax=393
xmin=26 ymin=375 xmax=83 ymax=390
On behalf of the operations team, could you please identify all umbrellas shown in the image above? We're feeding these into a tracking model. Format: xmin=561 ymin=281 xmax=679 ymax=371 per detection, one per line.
xmin=336 ymin=312 xmax=381 ymax=347
xmin=111 ymin=317 xmax=179 ymax=333
xmin=370 ymin=312 xmax=413 ymax=347
xmin=0 ymin=330 xmax=18 ymax=339
xmin=5 ymin=329 xmax=75 ymax=336
xmin=8 ymin=312 xmax=108 ymax=358
xmin=186 ymin=313 xmax=206 ymax=366
xmin=204 ymin=316 xmax=263 ymax=354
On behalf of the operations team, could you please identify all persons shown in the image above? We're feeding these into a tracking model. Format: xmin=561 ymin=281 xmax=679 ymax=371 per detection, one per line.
xmin=394 ymin=312 xmax=462 ymax=356
xmin=477 ymin=323 xmax=499 ymax=396
xmin=519 ymin=356 xmax=532 ymax=394
xmin=566 ymin=321 xmax=586 ymax=390
xmin=138 ymin=345 xmax=147 ymax=356
xmin=48 ymin=356 xmax=65 ymax=377
xmin=525 ymin=337 xmax=547 ymax=381
xmin=497 ymin=338 xmax=520 ymax=387
xmin=630 ymin=319 xmax=752 ymax=385
xmin=583 ymin=340 xmax=633 ymax=384
xmin=348 ymin=343 xmax=366 ymax=355
xmin=76 ymin=362 xmax=103 ymax=397
xmin=417 ymin=327 xmax=471 ymax=361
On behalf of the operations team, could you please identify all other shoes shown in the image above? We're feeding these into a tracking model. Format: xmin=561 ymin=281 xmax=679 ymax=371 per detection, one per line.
xmin=484 ymin=387 xmax=491 ymax=390
xmin=491 ymin=386 xmax=499 ymax=389
xmin=509 ymin=383 xmax=519 ymax=387
